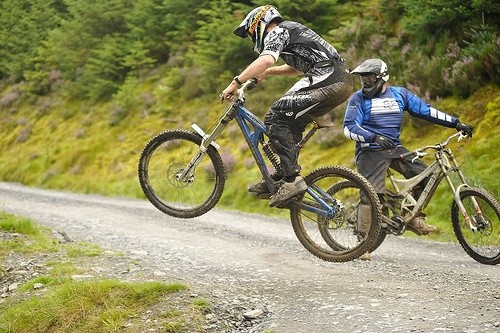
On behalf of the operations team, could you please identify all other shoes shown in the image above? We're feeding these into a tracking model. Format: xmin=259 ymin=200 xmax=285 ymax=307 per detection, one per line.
xmin=405 ymin=216 xmax=436 ymax=231
xmin=360 ymin=252 xmax=370 ymax=260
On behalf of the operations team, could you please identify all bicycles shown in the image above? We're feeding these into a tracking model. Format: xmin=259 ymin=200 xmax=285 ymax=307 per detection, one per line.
xmin=317 ymin=126 xmax=500 ymax=266
xmin=138 ymin=77 xmax=381 ymax=263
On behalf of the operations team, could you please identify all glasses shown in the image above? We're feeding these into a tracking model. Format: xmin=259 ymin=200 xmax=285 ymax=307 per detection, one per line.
xmin=360 ymin=76 xmax=376 ymax=87
xmin=247 ymin=32 xmax=253 ymax=42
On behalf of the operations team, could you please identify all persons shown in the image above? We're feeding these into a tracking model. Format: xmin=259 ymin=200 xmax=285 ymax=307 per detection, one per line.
xmin=219 ymin=5 xmax=352 ymax=207
xmin=343 ymin=58 xmax=473 ymax=259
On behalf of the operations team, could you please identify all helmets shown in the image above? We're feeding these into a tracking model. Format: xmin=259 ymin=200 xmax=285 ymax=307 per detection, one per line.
xmin=233 ymin=4 xmax=282 ymax=55
xmin=349 ymin=59 xmax=389 ymax=99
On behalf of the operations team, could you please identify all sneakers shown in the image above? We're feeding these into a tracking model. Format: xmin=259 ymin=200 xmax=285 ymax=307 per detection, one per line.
xmin=248 ymin=175 xmax=285 ymax=193
xmin=269 ymin=176 xmax=308 ymax=208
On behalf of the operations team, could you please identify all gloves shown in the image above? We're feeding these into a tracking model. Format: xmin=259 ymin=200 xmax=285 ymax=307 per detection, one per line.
xmin=456 ymin=121 xmax=472 ymax=137
xmin=375 ymin=135 xmax=394 ymax=150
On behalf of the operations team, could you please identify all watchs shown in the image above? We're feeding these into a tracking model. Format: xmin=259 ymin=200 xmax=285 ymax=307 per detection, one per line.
xmin=234 ymin=76 xmax=242 ymax=85
